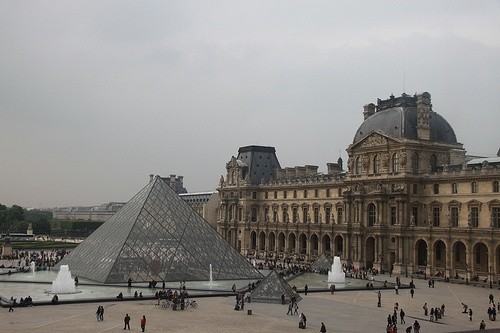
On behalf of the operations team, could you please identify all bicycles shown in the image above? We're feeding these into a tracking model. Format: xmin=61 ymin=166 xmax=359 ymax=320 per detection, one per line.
xmin=155 ymin=297 xmax=198 ymax=311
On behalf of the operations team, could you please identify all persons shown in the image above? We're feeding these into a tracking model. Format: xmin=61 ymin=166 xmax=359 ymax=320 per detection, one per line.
xmin=99 ymin=305 xmax=104 ymax=320
xmin=134 ymin=290 xmax=138 ymax=298
xmin=74 ymin=276 xmax=79 ymax=286
xmin=140 ymin=315 xmax=147 ymax=332
xmin=0 ymin=234 xmax=71 ymax=311
xmin=96 ymin=305 xmax=100 ymax=320
xmin=231 ymin=252 xmax=500 ymax=333
xmin=123 ymin=313 xmax=131 ymax=330
xmin=147 ymin=278 xmax=190 ymax=312
xmin=128 ymin=277 xmax=132 ymax=286
xmin=139 ymin=291 xmax=144 ymax=298
xmin=119 ymin=291 xmax=123 ymax=300
